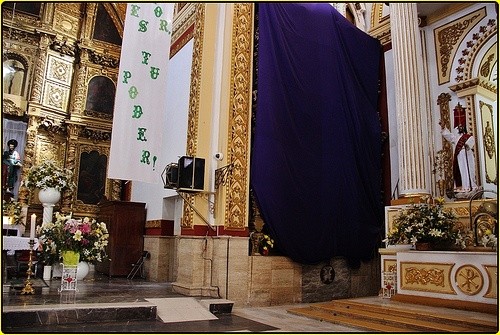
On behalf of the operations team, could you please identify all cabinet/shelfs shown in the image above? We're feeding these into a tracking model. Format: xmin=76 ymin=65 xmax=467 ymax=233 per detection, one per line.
xmin=94 ymin=201 xmax=145 ymax=278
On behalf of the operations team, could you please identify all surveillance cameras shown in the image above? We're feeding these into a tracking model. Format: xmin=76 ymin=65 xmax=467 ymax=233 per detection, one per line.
xmin=215 ymin=153 xmax=223 ymax=160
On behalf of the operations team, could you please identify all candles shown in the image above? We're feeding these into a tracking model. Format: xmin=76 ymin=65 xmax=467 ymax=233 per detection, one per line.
xmin=30 ymin=213 xmax=36 ymax=241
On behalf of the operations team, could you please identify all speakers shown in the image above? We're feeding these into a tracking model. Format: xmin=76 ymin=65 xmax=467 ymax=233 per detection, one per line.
xmin=177 ymin=157 xmax=205 ymax=190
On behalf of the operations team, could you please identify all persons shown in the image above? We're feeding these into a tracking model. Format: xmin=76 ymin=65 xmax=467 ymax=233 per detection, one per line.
xmin=442 ymin=123 xmax=477 ymax=190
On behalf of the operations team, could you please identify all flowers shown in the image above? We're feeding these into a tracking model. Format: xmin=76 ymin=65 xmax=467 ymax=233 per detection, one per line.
xmin=480 ymin=217 xmax=497 ymax=248
xmin=381 ymin=196 xmax=472 ymax=251
xmin=30 ymin=213 xmax=112 ymax=266
xmin=260 ymin=233 xmax=274 ymax=257
xmin=21 ymin=160 xmax=77 ymax=197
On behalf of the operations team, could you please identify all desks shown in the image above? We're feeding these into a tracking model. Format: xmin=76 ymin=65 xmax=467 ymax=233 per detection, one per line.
xmin=2 ymin=235 xmax=39 ymax=285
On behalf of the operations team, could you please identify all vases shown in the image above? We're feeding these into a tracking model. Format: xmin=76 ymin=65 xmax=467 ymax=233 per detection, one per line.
xmin=62 ymin=251 xmax=80 ymax=267
xmin=415 ymin=242 xmax=434 ymax=251
xmin=38 ymin=187 xmax=61 ymax=203
